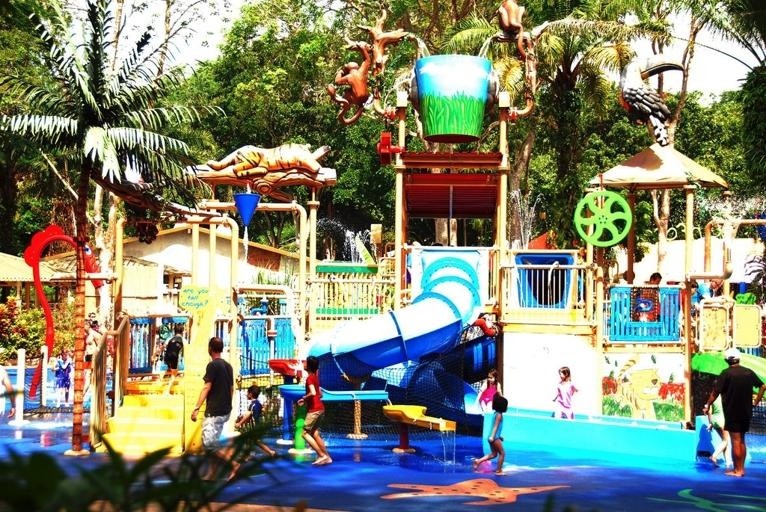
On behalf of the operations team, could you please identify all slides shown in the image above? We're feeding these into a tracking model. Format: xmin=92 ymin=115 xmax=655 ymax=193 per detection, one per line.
xmin=268 ymin=320 xmax=495 ymax=380
xmin=380 ymin=403 xmax=456 ymax=432
xmin=280 ymin=384 xmax=389 ymax=401
xmin=308 ymin=245 xmax=485 ymax=438
xmin=398 ymin=331 xmax=496 ymax=415
xmin=691 ymin=350 xmax=766 ymax=399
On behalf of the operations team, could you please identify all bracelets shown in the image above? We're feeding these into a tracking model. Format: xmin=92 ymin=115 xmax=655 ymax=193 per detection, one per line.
xmin=194 ymin=407 xmax=199 ymax=411
xmin=704 ymin=403 xmax=710 ymax=409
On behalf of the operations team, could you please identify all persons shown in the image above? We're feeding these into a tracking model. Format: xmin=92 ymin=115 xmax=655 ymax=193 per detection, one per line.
xmin=151 ymin=322 xmax=188 ymax=395
xmin=701 ymin=348 xmax=766 ymax=477
xmin=633 ymin=272 xmax=727 ymax=322
xmin=404 ymin=235 xmax=422 ymax=284
xmin=85 ymin=312 xmax=96 ymax=327
xmin=234 ymin=384 xmax=277 ymax=464
xmin=706 ymin=378 xmax=734 ymax=469
xmin=189 ymin=336 xmax=241 ymax=484
xmin=296 ymin=355 xmax=332 ymax=465
xmin=476 ymin=368 xmax=503 ymax=413
xmin=474 ymin=395 xmax=509 ymax=477
xmin=0 ymin=363 xmax=16 ymax=419
xmin=551 ymin=367 xmax=579 ymax=420
xmin=51 ymin=350 xmax=71 ymax=407
xmin=83 ymin=320 xmax=101 ymax=361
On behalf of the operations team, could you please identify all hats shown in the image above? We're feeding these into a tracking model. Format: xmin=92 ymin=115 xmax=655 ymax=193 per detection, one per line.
xmin=724 ymin=347 xmax=741 ymax=360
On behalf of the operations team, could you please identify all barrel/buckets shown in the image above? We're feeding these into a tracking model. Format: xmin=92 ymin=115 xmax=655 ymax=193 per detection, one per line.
xmin=416 ymin=54 xmax=491 ymax=142
